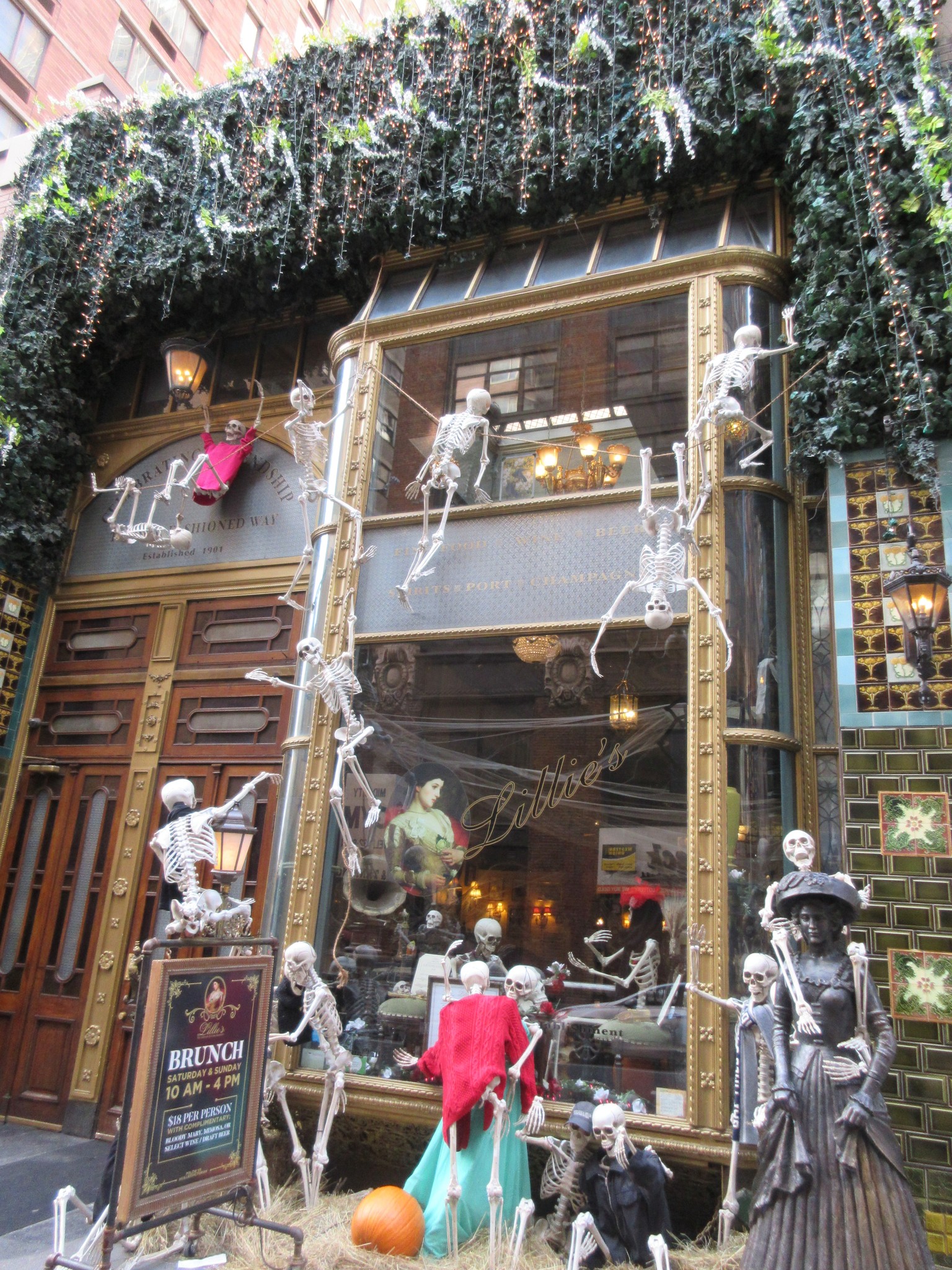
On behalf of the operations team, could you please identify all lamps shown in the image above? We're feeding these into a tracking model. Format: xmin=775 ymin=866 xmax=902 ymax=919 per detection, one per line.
xmin=209 ymin=798 xmax=259 ymax=896
xmin=535 ymin=362 xmax=631 ymax=495
xmin=607 ymin=630 xmax=639 ymax=730
xmin=883 ymin=522 xmax=952 ymax=703
xmin=159 ymin=330 xmax=212 ymax=409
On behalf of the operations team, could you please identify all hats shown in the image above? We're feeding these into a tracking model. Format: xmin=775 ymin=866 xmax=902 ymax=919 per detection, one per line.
xmin=563 ymin=1101 xmax=597 ymax=1132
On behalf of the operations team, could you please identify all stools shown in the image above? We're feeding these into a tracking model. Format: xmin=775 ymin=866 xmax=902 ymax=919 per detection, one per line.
xmin=595 ymin=1017 xmax=672 ymax=1105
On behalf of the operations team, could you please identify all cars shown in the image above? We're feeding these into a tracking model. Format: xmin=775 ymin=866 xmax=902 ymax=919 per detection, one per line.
xmin=549 ymin=980 xmax=694 ymax=1073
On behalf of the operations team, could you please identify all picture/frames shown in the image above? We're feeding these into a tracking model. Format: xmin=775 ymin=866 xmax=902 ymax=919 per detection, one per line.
xmin=424 ymin=975 xmax=503 ymax=1052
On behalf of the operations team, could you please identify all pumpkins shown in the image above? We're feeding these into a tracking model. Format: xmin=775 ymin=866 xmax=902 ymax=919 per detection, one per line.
xmin=350 ymin=1186 xmax=425 ymax=1259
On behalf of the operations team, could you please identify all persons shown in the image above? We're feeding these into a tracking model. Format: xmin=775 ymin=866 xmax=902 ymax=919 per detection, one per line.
xmin=91 ymin=379 xmax=265 ymax=550
xmin=680 ymin=307 xmax=800 ymax=555
xmin=52 ymin=768 xmax=673 ymax=1270
xmin=395 ymin=387 xmax=492 ymax=613
xmin=453 ymin=402 xmax=502 ymax=500
xmin=245 ymin=587 xmax=382 ymax=877
xmin=591 ymin=441 xmax=733 ymax=677
xmin=739 ymin=871 xmax=938 ymax=1270
xmin=685 ymin=828 xmax=871 ymax=1250
xmin=278 ymin=357 xmax=378 ymax=609
xmin=343 ymin=944 xmax=387 ymax=1063
